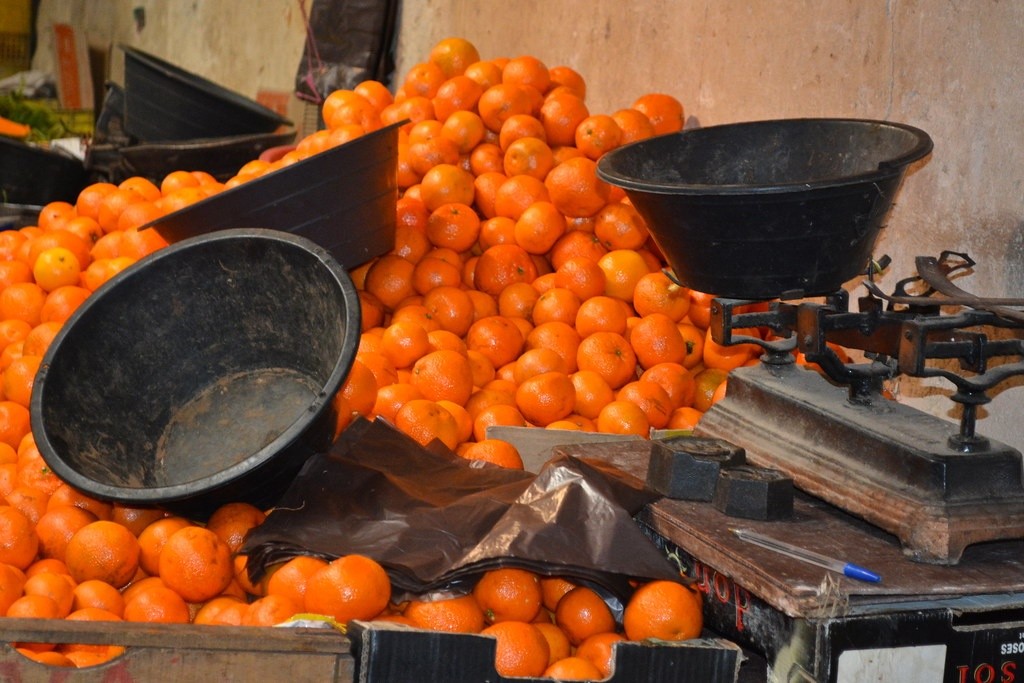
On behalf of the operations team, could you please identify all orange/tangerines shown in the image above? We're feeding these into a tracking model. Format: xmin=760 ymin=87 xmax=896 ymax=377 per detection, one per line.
xmin=1 ymin=36 xmax=853 ymax=683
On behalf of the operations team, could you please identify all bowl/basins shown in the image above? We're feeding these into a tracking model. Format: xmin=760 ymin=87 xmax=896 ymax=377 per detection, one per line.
xmin=119 ymin=131 xmax=296 ymax=182
xmin=30 ymin=225 xmax=355 ymax=525
xmin=137 ymin=119 xmax=414 ymax=274
xmin=598 ymin=117 xmax=934 ymax=300
xmin=120 ymin=37 xmax=293 ymax=140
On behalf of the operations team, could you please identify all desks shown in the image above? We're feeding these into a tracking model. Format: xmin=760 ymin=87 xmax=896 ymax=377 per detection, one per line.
xmin=553 ymin=437 xmax=1024 ymax=683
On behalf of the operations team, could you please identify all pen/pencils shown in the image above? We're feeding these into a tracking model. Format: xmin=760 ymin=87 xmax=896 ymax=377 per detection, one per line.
xmin=726 ymin=527 xmax=882 ymax=586
